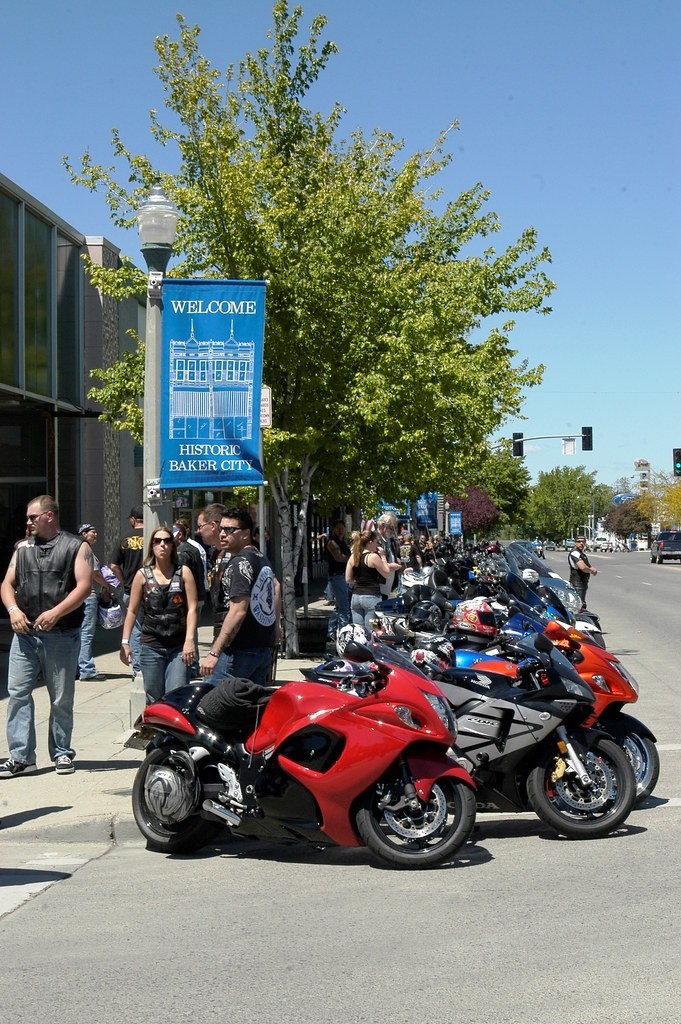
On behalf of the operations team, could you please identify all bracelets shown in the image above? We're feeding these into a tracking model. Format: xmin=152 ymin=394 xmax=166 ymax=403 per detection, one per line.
xmin=209 ymin=651 xmax=219 ymax=657
xmin=8 ymin=605 xmax=18 ymax=614
xmin=121 ymin=640 xmax=130 ymax=644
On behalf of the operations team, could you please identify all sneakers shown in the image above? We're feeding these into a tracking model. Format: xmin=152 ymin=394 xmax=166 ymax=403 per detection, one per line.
xmin=0 ymin=754 xmax=37 ymax=777
xmin=54 ymin=754 xmax=75 ymax=772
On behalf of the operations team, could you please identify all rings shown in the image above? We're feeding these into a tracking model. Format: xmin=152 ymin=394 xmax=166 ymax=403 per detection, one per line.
xmin=190 ymin=655 xmax=193 ymax=658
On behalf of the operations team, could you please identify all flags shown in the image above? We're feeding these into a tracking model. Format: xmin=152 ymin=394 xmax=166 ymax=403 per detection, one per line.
xmin=360 ymin=508 xmax=375 ymax=534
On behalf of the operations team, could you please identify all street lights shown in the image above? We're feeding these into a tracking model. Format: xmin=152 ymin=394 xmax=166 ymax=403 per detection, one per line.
xmin=134 ymin=174 xmax=179 ymax=729
xmin=577 ymin=496 xmax=595 ymax=542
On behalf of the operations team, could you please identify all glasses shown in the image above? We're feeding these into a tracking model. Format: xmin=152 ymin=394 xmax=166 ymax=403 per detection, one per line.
xmin=196 ymin=521 xmax=217 ymax=530
xmin=151 ymin=537 xmax=173 ymax=544
xmin=384 ymin=524 xmax=397 ymax=531
xmin=26 ymin=511 xmax=49 ymax=522
xmin=218 ymin=526 xmax=241 ymax=534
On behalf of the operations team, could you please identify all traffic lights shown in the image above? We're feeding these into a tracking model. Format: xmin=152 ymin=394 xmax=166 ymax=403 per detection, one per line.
xmin=673 ymin=447 xmax=681 ymax=477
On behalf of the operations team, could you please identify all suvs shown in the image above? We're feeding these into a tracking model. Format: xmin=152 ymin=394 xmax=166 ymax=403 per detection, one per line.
xmin=650 ymin=530 xmax=681 ymax=564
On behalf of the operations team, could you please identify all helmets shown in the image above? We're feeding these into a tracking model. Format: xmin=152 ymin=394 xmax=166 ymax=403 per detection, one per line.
xmin=335 ymin=623 xmax=369 ymax=656
xmin=97 ymin=604 xmax=123 ymax=629
xmin=486 ymin=545 xmax=501 ymax=555
xmin=453 ymin=600 xmax=497 ymax=638
xmin=408 ymin=599 xmax=442 ymax=632
xmin=410 ymin=634 xmax=457 ymax=674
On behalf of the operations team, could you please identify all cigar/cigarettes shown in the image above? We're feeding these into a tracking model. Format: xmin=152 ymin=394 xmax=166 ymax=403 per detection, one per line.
xmin=38 ymin=625 xmax=42 ymax=630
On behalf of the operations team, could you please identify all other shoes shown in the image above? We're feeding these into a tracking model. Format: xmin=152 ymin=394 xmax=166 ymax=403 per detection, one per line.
xmin=79 ymin=673 xmax=106 ymax=681
xmin=324 ymin=600 xmax=335 ymax=606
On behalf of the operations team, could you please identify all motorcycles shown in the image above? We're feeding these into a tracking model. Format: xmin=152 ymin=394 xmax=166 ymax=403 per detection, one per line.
xmin=122 ymin=622 xmax=479 ymax=871
xmin=297 ymin=537 xmax=660 ymax=840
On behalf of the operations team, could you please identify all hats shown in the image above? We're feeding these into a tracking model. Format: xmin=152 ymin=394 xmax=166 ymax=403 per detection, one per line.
xmin=77 ymin=523 xmax=96 ymax=535
xmin=128 ymin=505 xmax=143 ymax=518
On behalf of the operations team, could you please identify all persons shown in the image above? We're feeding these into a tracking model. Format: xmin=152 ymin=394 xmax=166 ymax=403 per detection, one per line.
xmin=568 ymin=534 xmax=597 ymax=609
xmin=0 ymin=496 xmax=93 ymax=777
xmin=316 ymin=512 xmax=462 ymax=652
xmin=65 ymin=522 xmax=110 ymax=682
xmin=532 ymin=537 xmax=542 ymax=544
xmin=108 ymin=499 xmax=281 ymax=756
